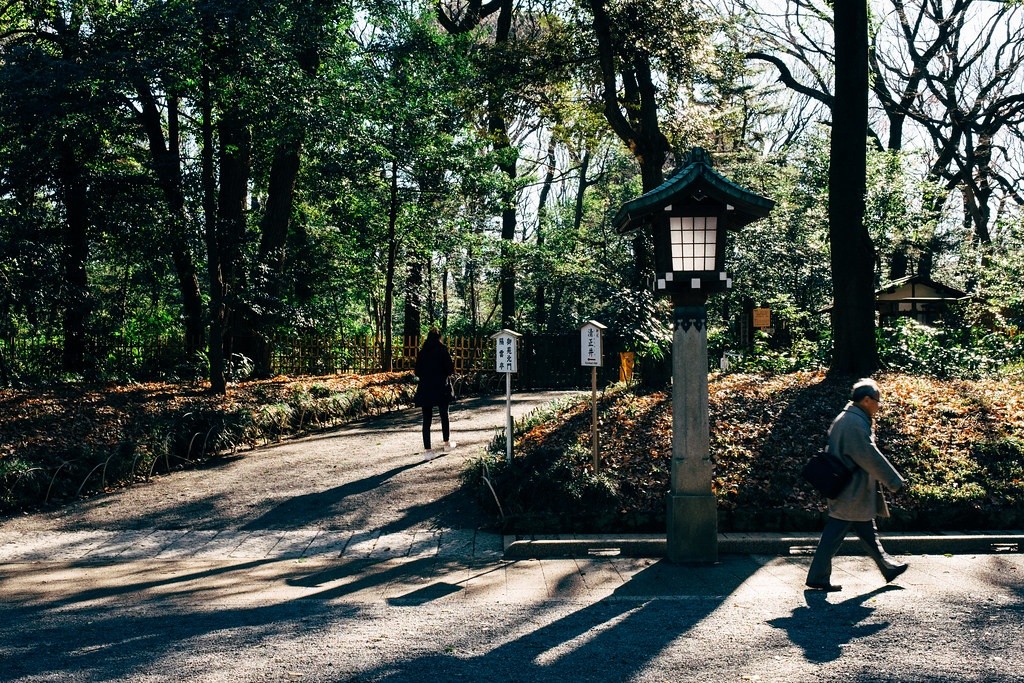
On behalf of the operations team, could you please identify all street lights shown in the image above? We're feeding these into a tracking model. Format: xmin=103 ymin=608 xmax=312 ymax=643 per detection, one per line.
xmin=612 ymin=145 xmax=778 ymax=563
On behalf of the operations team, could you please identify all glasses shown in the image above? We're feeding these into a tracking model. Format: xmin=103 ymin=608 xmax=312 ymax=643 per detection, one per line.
xmin=867 ymin=396 xmax=882 ymax=407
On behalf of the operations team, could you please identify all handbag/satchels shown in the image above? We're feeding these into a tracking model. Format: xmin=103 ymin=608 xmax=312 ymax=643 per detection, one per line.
xmin=803 ymin=453 xmax=853 ymax=497
xmin=438 ymin=375 xmax=455 ymax=402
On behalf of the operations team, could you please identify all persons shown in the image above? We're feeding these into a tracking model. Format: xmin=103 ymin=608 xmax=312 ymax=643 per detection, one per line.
xmin=804 ymin=378 xmax=910 ymax=591
xmin=414 ymin=327 xmax=456 ymax=452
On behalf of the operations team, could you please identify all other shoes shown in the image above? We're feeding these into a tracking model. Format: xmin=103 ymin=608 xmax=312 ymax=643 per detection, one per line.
xmin=805 ymin=583 xmax=842 ymax=591
xmin=885 ymin=564 xmax=908 ymax=583
xmin=444 ymin=441 xmax=456 ymax=452
